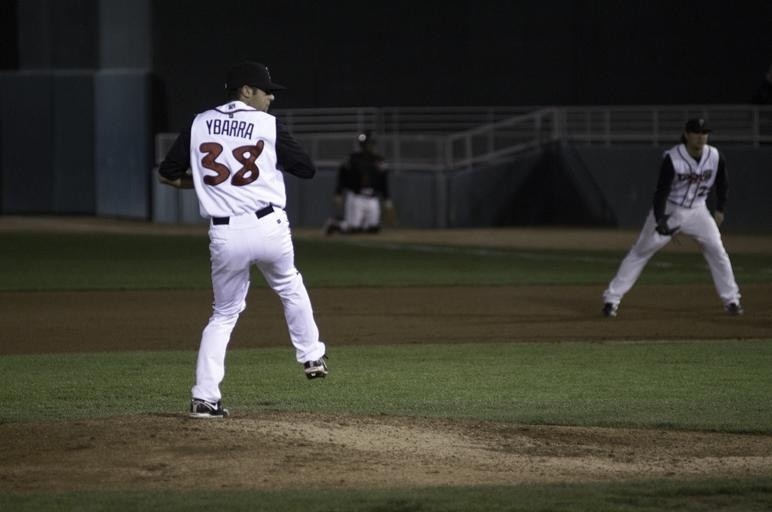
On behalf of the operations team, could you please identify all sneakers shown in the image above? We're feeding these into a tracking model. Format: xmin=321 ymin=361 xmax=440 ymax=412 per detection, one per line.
xmin=324 ymin=218 xmax=342 ymax=235
xmin=188 ymin=396 xmax=231 ymax=420
xmin=727 ymin=301 xmax=745 ymax=318
xmin=601 ymin=301 xmax=620 ymax=320
xmin=303 ymin=355 xmax=330 ymax=379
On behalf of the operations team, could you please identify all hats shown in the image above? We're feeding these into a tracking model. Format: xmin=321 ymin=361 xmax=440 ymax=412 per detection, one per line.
xmin=223 ymin=61 xmax=288 ymax=93
xmin=682 ymin=116 xmax=713 ymax=137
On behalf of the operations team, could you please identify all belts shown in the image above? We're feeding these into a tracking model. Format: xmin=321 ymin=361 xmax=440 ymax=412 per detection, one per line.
xmin=212 ymin=205 xmax=274 ymax=226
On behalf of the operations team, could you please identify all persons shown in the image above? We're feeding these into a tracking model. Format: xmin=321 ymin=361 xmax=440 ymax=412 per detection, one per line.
xmin=158 ymin=59 xmax=329 ymax=420
xmin=324 ymin=129 xmax=393 ymax=236
xmin=601 ymin=115 xmax=743 ymax=316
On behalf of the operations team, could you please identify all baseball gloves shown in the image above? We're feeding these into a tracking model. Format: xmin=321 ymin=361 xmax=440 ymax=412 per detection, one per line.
xmin=656 ymin=212 xmax=680 ymax=235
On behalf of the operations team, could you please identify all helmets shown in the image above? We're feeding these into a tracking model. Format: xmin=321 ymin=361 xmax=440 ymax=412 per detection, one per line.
xmin=356 ymin=129 xmax=377 ymax=147
